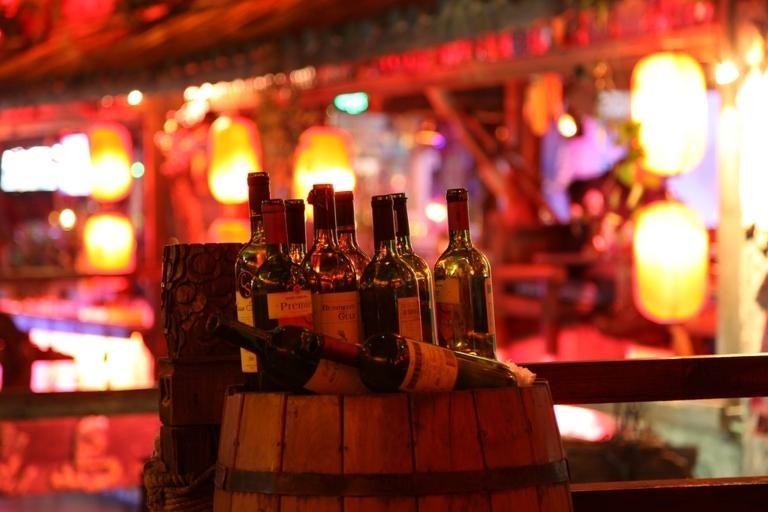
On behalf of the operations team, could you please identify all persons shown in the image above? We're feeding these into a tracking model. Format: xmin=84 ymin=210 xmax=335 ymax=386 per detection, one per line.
xmin=553 ymin=179 xmax=617 ymax=315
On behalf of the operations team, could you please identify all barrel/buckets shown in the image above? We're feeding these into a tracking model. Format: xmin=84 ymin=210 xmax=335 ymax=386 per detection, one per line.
xmin=213 ymin=377 xmax=572 ymax=512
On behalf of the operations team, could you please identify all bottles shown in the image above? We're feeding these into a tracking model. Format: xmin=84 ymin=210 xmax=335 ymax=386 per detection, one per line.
xmin=304 ymin=183 xmax=357 ymax=360
xmin=384 ymin=192 xmax=438 ymax=347
xmin=249 ymin=195 xmax=311 ymax=373
xmin=284 ymin=199 xmax=307 ymax=262
xmin=233 ymin=171 xmax=271 ymax=375
xmin=334 ymin=189 xmax=371 ymax=273
xmin=206 ymin=314 xmax=365 ymax=393
xmin=360 ymin=195 xmax=424 ymax=346
xmin=434 ymin=187 xmax=498 ymax=361
xmin=300 ymin=332 xmax=510 ymax=391
